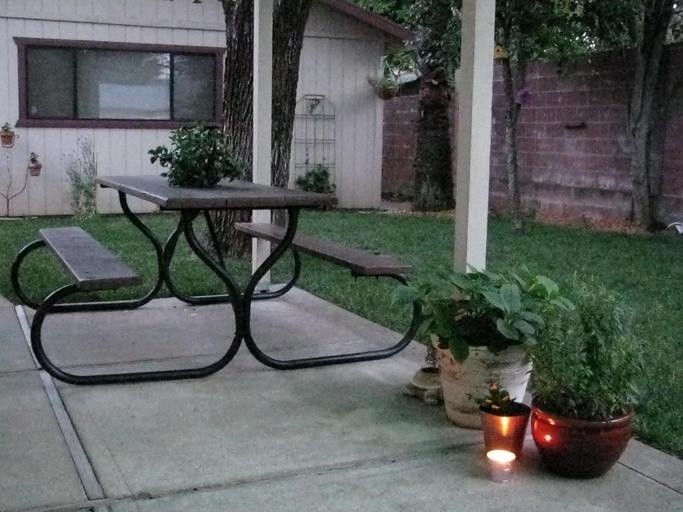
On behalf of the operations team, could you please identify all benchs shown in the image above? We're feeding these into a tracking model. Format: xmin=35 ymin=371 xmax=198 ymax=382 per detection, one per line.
xmin=10 ymin=226 xmax=142 ymax=386
xmin=233 ymin=222 xmax=418 ymax=369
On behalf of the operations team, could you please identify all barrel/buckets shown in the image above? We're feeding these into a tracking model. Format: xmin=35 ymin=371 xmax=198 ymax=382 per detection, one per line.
xmin=479 ymin=401 xmax=532 ymax=462
xmin=433 ymin=335 xmax=534 ymax=430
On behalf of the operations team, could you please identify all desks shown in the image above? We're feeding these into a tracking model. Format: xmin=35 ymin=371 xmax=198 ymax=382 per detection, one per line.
xmin=93 ymin=173 xmax=332 ymax=383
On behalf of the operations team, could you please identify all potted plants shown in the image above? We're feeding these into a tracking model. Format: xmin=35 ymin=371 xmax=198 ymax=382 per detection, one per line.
xmin=0 ymin=120 xmax=15 ymax=146
xmin=27 ymin=152 xmax=43 ymax=176
xmin=147 ymin=119 xmax=247 ymax=188
xmin=373 ymin=77 xmax=398 ymax=100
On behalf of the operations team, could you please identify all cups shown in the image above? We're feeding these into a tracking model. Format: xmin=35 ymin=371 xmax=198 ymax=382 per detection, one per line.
xmin=485 ymin=449 xmax=516 ymax=482
